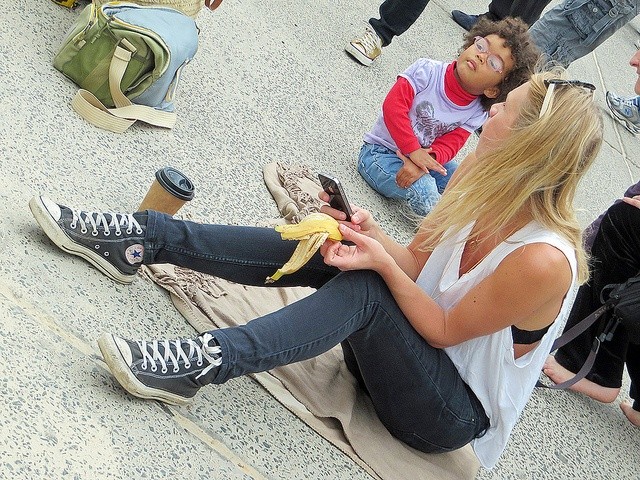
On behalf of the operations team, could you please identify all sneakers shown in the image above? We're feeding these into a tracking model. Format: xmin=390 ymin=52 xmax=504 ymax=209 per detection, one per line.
xmin=452 ymin=10 xmax=483 ymax=33
xmin=97 ymin=331 xmax=223 ymax=408
xmin=345 ymin=19 xmax=383 ymax=67
xmin=29 ymin=193 xmax=149 ymax=286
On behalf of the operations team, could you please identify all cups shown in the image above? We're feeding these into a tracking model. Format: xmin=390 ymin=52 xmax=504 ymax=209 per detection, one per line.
xmin=137 ymin=167 xmax=194 ymax=216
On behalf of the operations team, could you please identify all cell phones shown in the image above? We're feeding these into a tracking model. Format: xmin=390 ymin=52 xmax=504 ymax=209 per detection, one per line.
xmin=318 ymin=172 xmax=353 ymax=222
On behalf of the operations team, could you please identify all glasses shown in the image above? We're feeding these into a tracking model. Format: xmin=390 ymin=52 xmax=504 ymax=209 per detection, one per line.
xmin=472 ymin=36 xmax=505 ymax=86
xmin=538 ymin=79 xmax=597 ymax=120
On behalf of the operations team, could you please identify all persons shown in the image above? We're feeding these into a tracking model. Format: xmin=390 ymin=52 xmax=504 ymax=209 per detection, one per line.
xmin=542 ymin=38 xmax=640 ymax=429
xmin=345 ymin=0 xmax=430 ymax=66
xmin=451 ymin=0 xmax=552 ymax=33
xmin=524 ymin=1 xmax=640 ymax=74
xmin=29 ymin=70 xmax=604 ymax=471
xmin=358 ymin=16 xmax=537 ymax=217
xmin=630 ymin=50 xmax=640 ymax=96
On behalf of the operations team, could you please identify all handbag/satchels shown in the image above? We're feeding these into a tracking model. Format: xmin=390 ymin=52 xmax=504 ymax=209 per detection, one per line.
xmin=52 ymin=0 xmax=200 ymax=134
xmin=599 ymin=270 xmax=639 ymax=345
